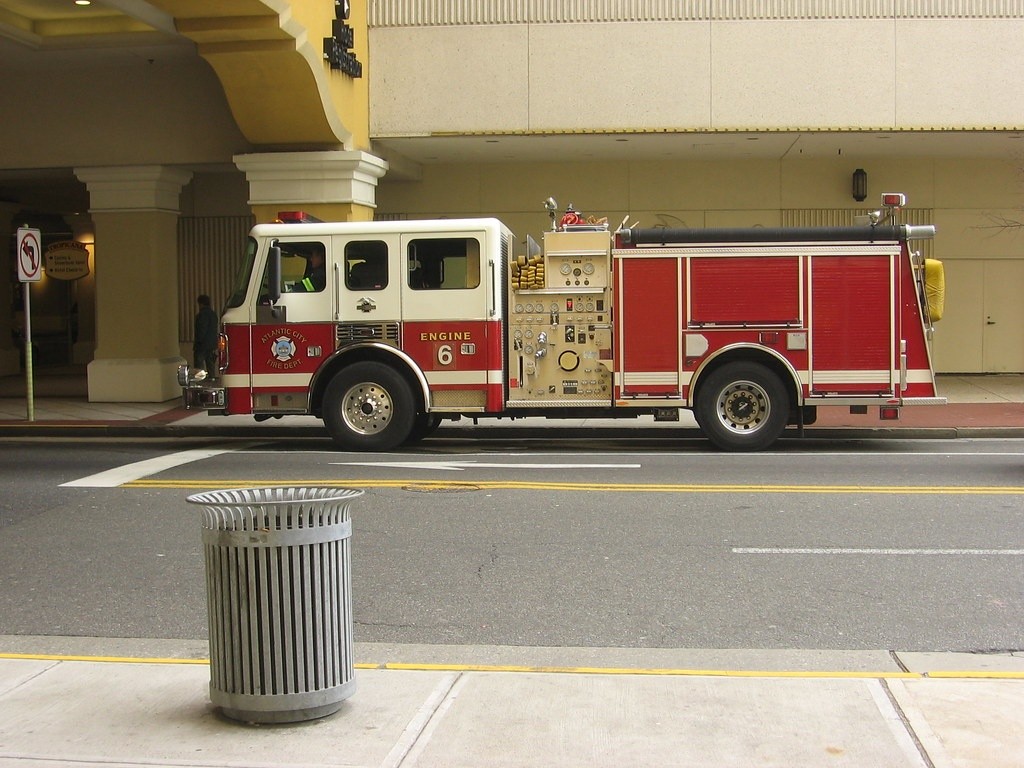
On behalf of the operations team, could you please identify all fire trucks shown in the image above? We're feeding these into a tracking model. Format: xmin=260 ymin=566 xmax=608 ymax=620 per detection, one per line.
xmin=175 ymin=192 xmax=947 ymax=453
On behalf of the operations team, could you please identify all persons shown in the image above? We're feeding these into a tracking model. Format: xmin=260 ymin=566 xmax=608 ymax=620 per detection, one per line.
xmin=193 ymin=295 xmax=218 ymax=377
xmin=287 ymin=250 xmax=326 ymax=293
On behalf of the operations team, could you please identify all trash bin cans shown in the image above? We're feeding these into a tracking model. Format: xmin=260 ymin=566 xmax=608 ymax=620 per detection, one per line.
xmin=185 ymin=486 xmax=366 ymax=724
